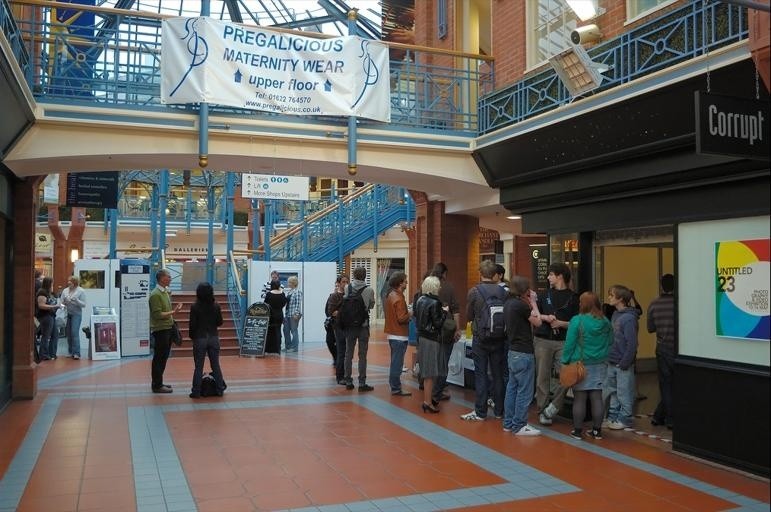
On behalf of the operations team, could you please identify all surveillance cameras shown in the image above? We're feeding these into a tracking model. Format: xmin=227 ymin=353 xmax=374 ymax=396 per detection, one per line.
xmin=570 ymin=24 xmax=600 ymax=46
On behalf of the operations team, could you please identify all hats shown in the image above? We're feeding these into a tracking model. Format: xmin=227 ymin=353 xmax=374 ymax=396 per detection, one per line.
xmin=354 ymin=267 xmax=366 ymax=280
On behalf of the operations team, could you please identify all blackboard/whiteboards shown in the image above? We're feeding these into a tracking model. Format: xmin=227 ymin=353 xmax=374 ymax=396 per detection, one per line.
xmin=239 ymin=302 xmax=270 ymax=356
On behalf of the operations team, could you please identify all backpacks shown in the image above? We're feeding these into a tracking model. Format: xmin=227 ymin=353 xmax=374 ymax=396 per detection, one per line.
xmin=476 ymin=284 xmax=507 ymax=339
xmin=342 ymin=284 xmax=370 ymax=327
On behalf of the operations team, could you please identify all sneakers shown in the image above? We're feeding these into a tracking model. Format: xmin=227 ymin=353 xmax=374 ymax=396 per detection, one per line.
xmin=539 ymin=413 xmax=553 ymax=425
xmin=504 ymin=423 xmax=541 ymax=436
xmin=154 ymin=384 xmax=173 ymax=393
xmin=64 ymin=353 xmax=79 ymax=360
xmin=189 ymin=393 xmax=200 ymax=397
xmin=543 ymin=403 xmax=560 ymax=419
xmin=585 ymin=426 xmax=602 ymax=440
xmin=460 ymin=410 xmax=486 ymax=421
xmin=569 ymin=427 xmax=583 ymax=440
xmin=339 ymin=380 xmax=354 ymax=390
xmin=601 ymin=419 xmax=632 ymax=430
xmin=358 ymin=384 xmax=374 ymax=392
xmin=392 ymin=390 xmax=411 ymax=396
xmin=217 ymin=390 xmax=222 ymax=396
xmin=280 ymin=347 xmax=296 ymax=353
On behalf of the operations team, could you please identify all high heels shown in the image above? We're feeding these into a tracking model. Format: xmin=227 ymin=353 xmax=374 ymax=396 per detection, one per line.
xmin=423 ymin=402 xmax=440 ymax=413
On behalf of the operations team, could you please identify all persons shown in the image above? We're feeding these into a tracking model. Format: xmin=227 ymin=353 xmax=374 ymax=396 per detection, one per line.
xmin=261 ymin=269 xmax=284 ymax=302
xmin=189 ymin=283 xmax=227 ymax=398
xmin=35 ymin=267 xmax=44 ymax=341
xmin=281 ymin=275 xmax=302 ymax=353
xmin=381 ymin=263 xmax=460 ymax=413
xmin=323 ymin=265 xmax=376 ymax=392
xmin=148 ymin=269 xmax=183 ymax=393
xmin=36 ymin=277 xmax=62 ymax=359
xmin=60 ymin=276 xmax=86 ymax=359
xmin=264 ymin=278 xmax=287 ymax=355
xmin=460 ymin=260 xmax=677 ymax=441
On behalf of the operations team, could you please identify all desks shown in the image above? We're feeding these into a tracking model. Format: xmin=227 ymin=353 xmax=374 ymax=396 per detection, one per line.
xmin=446 ymin=329 xmax=593 ymax=423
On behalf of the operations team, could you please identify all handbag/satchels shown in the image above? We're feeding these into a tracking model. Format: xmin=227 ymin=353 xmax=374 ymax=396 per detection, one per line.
xmin=559 ymin=361 xmax=585 ymax=387
xmin=172 ymin=322 xmax=181 ymax=346
xmin=201 ymin=374 xmax=217 ymax=397
xmin=441 ymin=319 xmax=456 ymax=336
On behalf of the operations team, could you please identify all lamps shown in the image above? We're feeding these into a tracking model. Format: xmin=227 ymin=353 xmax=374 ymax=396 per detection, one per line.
xmin=71 ymin=247 xmax=79 ymax=262
xmin=548 ymin=45 xmax=615 ymax=99
xmin=565 ymin=0 xmax=607 ymax=22
